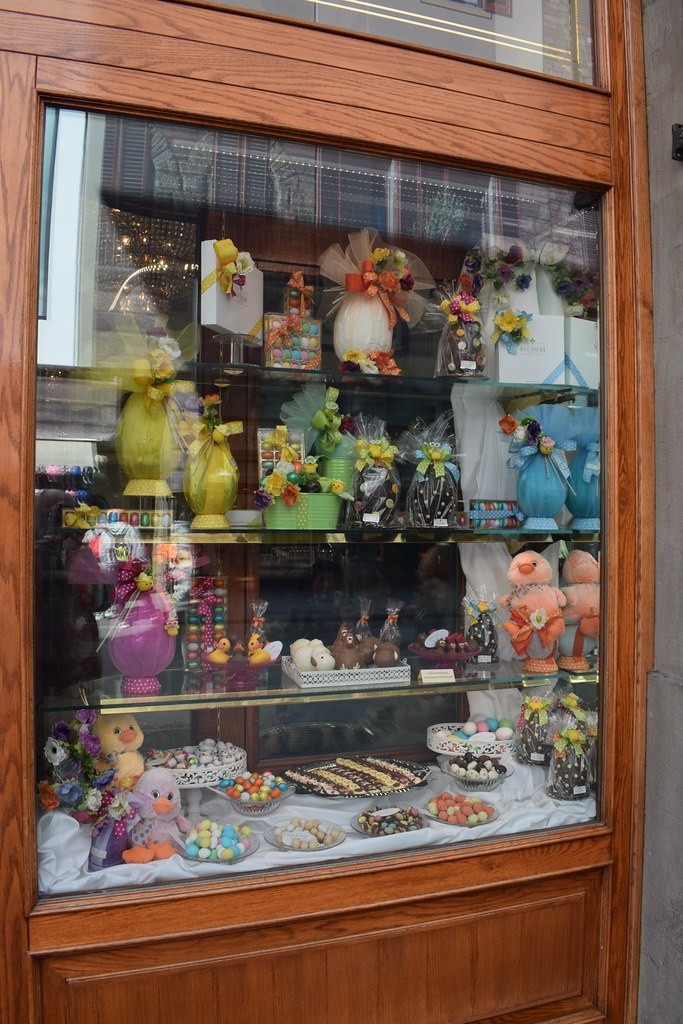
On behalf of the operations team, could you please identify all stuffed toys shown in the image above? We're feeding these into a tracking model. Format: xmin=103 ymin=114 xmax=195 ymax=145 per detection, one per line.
xmin=83 ymin=710 xmax=195 ymax=866
xmin=500 ymin=549 xmax=599 ymax=674
xmin=288 ymin=622 xmax=401 ymax=672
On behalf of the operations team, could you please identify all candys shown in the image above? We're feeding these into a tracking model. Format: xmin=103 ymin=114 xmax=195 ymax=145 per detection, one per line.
xmin=452 ymin=502 xmax=520 ymax=527
xmin=147 ymin=737 xmax=245 ymax=769
xmin=80 ymin=511 xmax=172 ymax=529
xmin=272 ymin=289 xmax=318 ymax=369
xmin=261 ymin=432 xmax=304 ymax=483
xmin=275 ymin=816 xmax=340 ymax=849
xmin=187 ymin=580 xmax=225 ymax=667
xmin=219 ymin=773 xmax=287 ymax=812
xmin=358 ymin=804 xmax=424 ymax=836
xmin=185 ymin=819 xmax=252 ymax=861
xmin=427 ymin=793 xmax=494 ymax=824
xmin=435 ymin=717 xmax=515 ymax=754
xmin=449 ymin=752 xmax=507 ymax=779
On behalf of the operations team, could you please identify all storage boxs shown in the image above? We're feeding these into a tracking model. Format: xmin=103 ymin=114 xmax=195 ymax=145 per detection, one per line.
xmin=494 ymin=314 xmax=565 ymax=384
xmin=201 ymin=240 xmax=264 ymax=349
xmin=283 ymin=285 xmax=313 ymax=316
xmin=566 ymin=318 xmax=599 ymax=388
xmin=256 ymin=427 xmax=305 ymax=478
xmin=279 ymin=655 xmax=410 ymax=688
xmin=264 ymin=312 xmax=323 ymax=371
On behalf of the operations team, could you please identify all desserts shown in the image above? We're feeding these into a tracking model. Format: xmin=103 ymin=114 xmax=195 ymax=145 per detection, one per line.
xmin=284 ymin=756 xmax=429 ymax=795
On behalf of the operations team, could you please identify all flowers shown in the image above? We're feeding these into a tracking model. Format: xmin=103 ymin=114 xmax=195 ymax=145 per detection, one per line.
xmin=36 ymin=710 xmax=131 ymax=837
xmin=232 ymin=251 xmax=254 ymax=286
xmin=251 ymin=470 xmax=301 ymax=511
xmin=139 ymin=543 xmax=191 ymax=570
xmin=530 ymin=607 xmax=549 ymax=630
xmin=465 ymin=245 xmax=541 ymax=296
xmin=145 ymin=326 xmax=181 ymax=385
xmin=497 ymin=412 xmax=556 ymax=447
xmin=185 ymin=392 xmax=223 ymax=435
xmin=338 ymin=350 xmax=402 ymax=375
xmin=541 ymin=245 xmax=600 ymax=318
xmin=368 ymin=247 xmax=414 ymax=293
xmin=34 ymin=362 xmax=608 ymax=893
xmin=493 ymin=308 xmax=533 ymax=341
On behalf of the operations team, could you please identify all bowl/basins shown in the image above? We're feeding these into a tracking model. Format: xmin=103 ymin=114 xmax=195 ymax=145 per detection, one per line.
xmin=440 ymin=761 xmax=515 ymax=792
xmin=216 ymin=778 xmax=296 ymax=818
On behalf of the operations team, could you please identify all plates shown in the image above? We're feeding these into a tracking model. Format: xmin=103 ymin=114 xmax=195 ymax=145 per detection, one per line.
xmin=350 ymin=811 xmax=431 ymax=837
xmin=263 ymin=818 xmax=347 ymax=852
xmin=173 ymin=831 xmax=261 ymax=865
xmin=422 ymin=801 xmax=501 ymax=827
